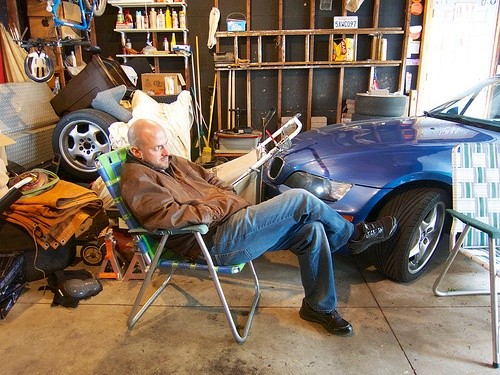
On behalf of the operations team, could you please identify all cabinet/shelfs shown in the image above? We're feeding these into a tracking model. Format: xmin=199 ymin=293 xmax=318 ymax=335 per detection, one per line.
xmin=106 ymin=0 xmax=191 ymax=59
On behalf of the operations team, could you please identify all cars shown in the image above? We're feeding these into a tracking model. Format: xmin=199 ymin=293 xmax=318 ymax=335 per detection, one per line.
xmin=259 ymin=75 xmax=499 ymax=282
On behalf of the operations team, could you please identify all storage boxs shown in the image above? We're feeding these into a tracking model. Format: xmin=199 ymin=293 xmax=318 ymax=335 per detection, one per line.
xmin=27 ymin=0 xmax=83 ymax=40
xmin=141 ymin=72 xmax=186 ymax=95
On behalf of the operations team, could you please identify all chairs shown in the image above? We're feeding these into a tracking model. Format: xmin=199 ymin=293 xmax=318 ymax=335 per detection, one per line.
xmin=431 ymin=142 xmax=500 ymax=368
xmin=94 ymin=144 xmax=261 ymax=343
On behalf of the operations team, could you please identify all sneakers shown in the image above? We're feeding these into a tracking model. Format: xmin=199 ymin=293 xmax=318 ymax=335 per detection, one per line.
xmin=299 ymin=298 xmax=353 ymax=336
xmin=348 ymin=216 xmax=398 ymax=255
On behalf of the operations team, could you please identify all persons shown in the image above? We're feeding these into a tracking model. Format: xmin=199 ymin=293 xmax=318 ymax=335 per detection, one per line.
xmin=121 ymin=119 xmax=397 ymax=335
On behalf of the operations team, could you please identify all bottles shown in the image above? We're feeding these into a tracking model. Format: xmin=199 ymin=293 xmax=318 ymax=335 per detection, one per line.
xmin=163 ymin=37 xmax=169 ymax=51
xmin=117 ymin=5 xmax=185 ymax=29
xmin=170 ymin=33 xmax=176 ymax=50
xmin=70 ymin=51 xmax=77 ymax=68
